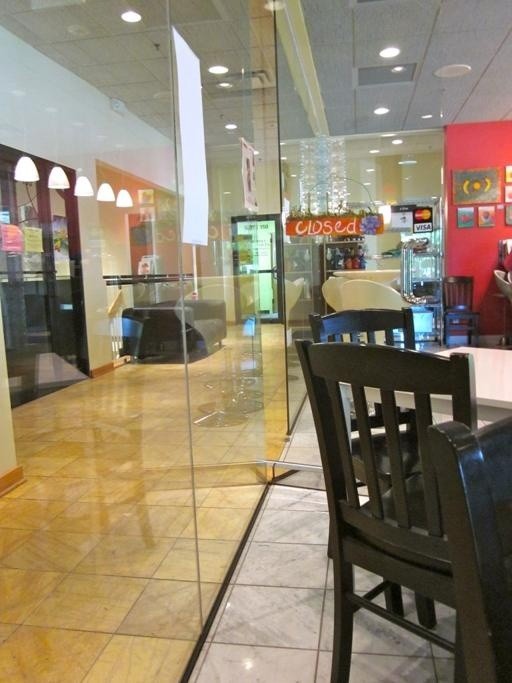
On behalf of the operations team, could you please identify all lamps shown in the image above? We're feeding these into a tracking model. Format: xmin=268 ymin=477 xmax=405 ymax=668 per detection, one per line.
xmin=13 ymin=155 xmax=134 ymax=209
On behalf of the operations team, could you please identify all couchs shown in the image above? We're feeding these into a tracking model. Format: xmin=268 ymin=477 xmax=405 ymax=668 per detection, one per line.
xmin=120 ymin=299 xmax=228 ymax=363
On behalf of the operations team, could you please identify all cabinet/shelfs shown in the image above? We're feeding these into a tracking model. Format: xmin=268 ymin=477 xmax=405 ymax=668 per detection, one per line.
xmin=322 ymin=238 xmax=365 ymax=315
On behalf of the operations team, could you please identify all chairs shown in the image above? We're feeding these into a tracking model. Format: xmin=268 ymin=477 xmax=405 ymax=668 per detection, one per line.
xmin=340 ymin=279 xmax=411 ymax=345
xmin=321 ymin=276 xmax=357 ymax=344
xmin=493 ymin=269 xmax=512 ymax=349
xmin=308 ymin=306 xmax=417 ymax=618
xmin=441 ymin=275 xmax=480 ymax=347
xmin=292 ymin=337 xmax=478 ymax=682
xmin=426 ymin=415 xmax=511 ymax=682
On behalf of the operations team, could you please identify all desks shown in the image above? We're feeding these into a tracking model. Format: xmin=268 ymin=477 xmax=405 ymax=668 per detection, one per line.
xmin=333 ymin=270 xmax=403 ymax=286
xmin=340 ymin=345 xmax=512 ymax=426
